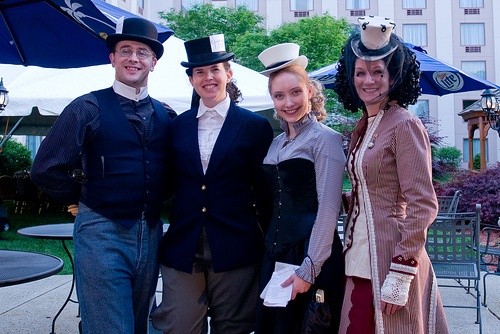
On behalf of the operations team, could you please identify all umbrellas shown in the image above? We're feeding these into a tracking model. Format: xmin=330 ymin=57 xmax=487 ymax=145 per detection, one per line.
xmin=308 ymin=45 xmax=500 ymax=96
xmin=0 ymin=36 xmax=275 ymax=118
xmin=0 ymin=0 xmax=175 ymax=69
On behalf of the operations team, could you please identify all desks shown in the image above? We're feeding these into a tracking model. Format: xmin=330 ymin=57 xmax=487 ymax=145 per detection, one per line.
xmin=17 ymin=223 xmax=170 ymax=334
xmin=0 ymin=249 xmax=64 ymax=287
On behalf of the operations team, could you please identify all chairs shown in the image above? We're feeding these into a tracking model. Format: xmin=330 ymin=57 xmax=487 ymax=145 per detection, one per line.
xmin=337 ymin=190 xmax=500 ymax=334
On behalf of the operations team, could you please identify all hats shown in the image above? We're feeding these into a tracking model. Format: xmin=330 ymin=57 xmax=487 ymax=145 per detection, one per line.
xmin=350 ymin=15 xmax=398 ymax=62
xmin=180 ymin=34 xmax=235 ymax=68
xmin=106 ymin=17 xmax=164 ymax=61
xmin=258 ymin=43 xmax=309 ymax=77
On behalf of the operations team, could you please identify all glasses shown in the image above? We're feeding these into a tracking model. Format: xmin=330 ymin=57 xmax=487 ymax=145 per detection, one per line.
xmin=114 ymin=47 xmax=153 ymax=60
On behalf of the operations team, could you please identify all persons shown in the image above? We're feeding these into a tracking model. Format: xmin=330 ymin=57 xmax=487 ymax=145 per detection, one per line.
xmin=152 ymin=34 xmax=273 ymax=333
xmin=332 ymin=17 xmax=451 ymax=333
xmin=32 ymin=17 xmax=181 ymax=333
xmin=254 ymin=43 xmax=346 ymax=333
